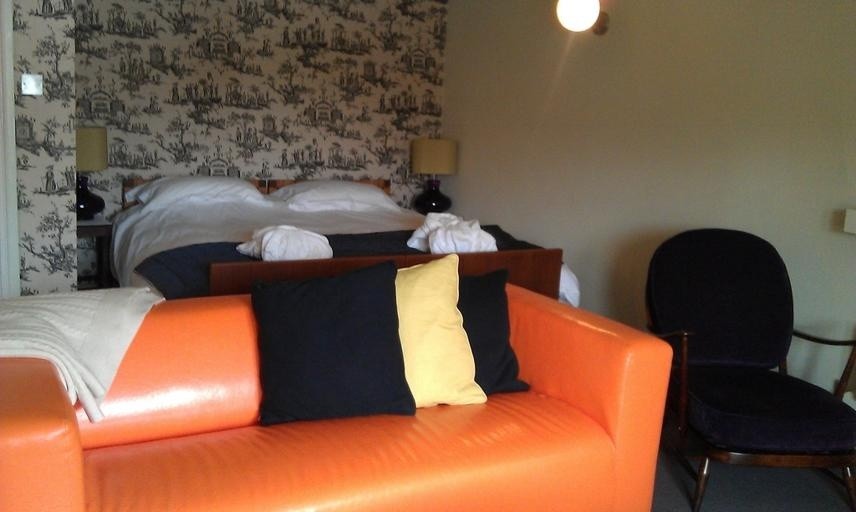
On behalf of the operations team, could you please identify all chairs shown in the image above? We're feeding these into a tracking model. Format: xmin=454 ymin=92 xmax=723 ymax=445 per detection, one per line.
xmin=647 ymin=228 xmax=856 ymax=512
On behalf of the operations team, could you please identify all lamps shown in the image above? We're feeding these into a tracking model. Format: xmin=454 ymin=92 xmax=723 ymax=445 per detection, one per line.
xmin=76 ymin=125 xmax=109 ymax=220
xmin=556 ymin=0 xmax=610 ymax=36
xmin=409 ymin=139 xmax=459 ymax=214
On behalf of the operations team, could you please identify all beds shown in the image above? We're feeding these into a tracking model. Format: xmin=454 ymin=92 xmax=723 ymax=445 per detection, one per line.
xmin=111 ymin=170 xmax=580 ymax=308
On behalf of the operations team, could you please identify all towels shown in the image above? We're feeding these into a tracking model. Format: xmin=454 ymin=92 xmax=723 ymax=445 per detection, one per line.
xmin=236 ymin=225 xmax=334 ymax=262
xmin=133 ymin=225 xmax=565 ymax=300
xmin=408 ymin=211 xmax=498 ymax=254
xmin=0 ymin=282 xmax=165 ymax=424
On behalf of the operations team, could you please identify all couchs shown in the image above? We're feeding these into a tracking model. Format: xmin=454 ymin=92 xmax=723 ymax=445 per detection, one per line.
xmin=0 ymin=276 xmax=673 ymax=510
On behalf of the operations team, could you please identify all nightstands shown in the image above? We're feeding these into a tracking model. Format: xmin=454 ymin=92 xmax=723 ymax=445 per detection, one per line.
xmin=76 ymin=218 xmax=114 ymax=289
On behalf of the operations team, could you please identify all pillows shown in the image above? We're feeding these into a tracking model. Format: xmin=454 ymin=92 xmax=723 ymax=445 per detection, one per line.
xmin=458 ymin=268 xmax=530 ymax=395
xmin=395 ymin=253 xmax=488 ymax=408
xmin=124 ymin=175 xmax=270 ymax=206
xmin=249 ymin=259 xmax=416 ymax=426
xmin=272 ymin=180 xmax=402 ymax=212
